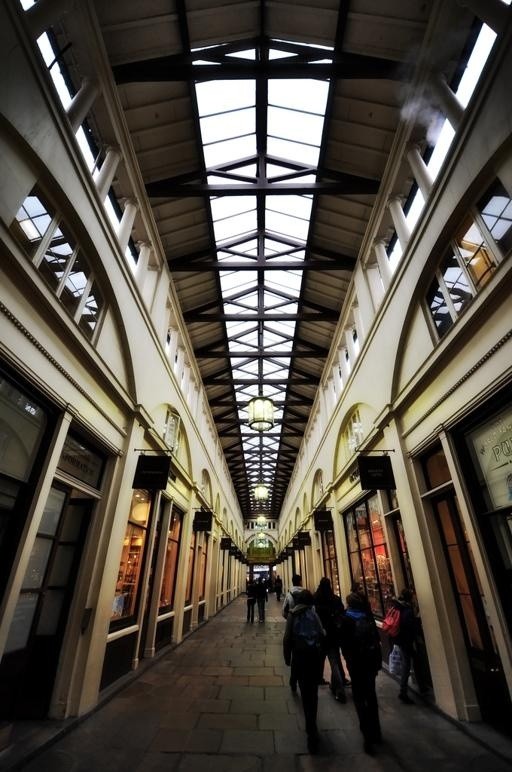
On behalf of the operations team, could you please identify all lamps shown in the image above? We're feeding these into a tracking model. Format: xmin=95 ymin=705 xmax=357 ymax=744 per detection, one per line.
xmin=246 ymin=392 xmax=274 ymax=432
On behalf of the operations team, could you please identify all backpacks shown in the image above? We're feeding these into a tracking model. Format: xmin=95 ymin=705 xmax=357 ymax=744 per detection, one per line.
xmin=380 ymin=602 xmax=402 ymax=639
xmin=292 ymin=611 xmax=324 ymax=658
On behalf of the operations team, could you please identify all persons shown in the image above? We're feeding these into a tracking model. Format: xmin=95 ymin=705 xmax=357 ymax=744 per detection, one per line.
xmin=255 ymin=577 xmax=268 ymax=623
xmin=392 ymin=587 xmax=430 ymax=704
xmin=280 ymin=574 xmax=399 ymax=705
xmin=245 ymin=578 xmax=257 ymax=623
xmin=254 ymin=573 xmax=283 ymax=602
xmin=336 ymin=593 xmax=385 ymax=757
xmin=279 ymin=588 xmax=328 ymax=757
